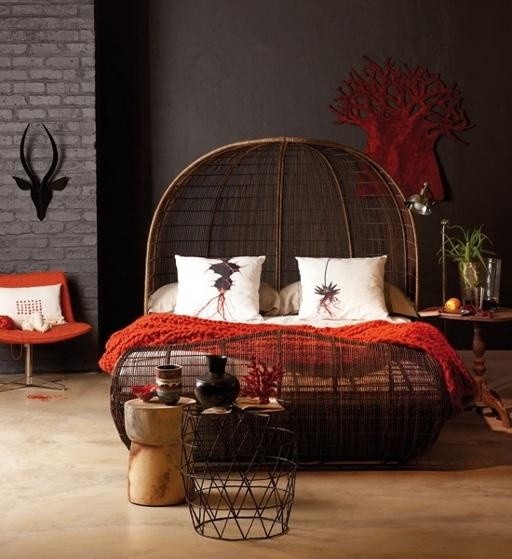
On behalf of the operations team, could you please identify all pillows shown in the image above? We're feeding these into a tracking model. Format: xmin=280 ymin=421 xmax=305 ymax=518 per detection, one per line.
xmin=279 ymin=278 xmax=418 ymax=317
xmin=295 ymin=254 xmax=394 ymax=323
xmin=0 ymin=283 xmax=69 ymax=329
xmin=172 ymin=253 xmax=266 ymax=324
xmin=147 ymin=282 xmax=280 ymax=317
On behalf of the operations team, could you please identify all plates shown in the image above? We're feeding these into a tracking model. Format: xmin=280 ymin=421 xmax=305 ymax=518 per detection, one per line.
xmin=439 ymin=309 xmax=471 ymax=316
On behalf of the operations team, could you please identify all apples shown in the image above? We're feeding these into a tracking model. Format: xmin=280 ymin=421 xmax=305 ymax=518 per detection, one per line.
xmin=444 ymin=298 xmax=460 ymax=311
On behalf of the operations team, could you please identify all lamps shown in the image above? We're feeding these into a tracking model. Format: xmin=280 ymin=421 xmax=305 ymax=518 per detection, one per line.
xmin=404 ymin=181 xmax=449 ymax=306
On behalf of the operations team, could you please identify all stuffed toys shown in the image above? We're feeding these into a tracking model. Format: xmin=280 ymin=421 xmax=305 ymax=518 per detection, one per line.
xmin=17 ymin=312 xmax=53 ymax=333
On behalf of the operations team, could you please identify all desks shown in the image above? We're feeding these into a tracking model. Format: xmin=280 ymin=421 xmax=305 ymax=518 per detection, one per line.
xmin=178 ymin=397 xmax=298 ymax=541
xmin=438 ymin=306 xmax=512 ymax=428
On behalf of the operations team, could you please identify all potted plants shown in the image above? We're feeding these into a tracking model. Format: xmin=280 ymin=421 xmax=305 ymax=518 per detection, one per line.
xmin=436 ymin=220 xmax=501 ymax=312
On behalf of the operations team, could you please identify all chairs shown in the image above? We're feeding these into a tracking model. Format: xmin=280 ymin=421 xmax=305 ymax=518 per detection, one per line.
xmin=0 ymin=271 xmax=92 ymax=392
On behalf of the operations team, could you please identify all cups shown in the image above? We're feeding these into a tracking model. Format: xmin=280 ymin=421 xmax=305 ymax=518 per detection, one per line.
xmin=459 ymin=258 xmax=501 ymax=312
xmin=156 ymin=364 xmax=184 ymax=406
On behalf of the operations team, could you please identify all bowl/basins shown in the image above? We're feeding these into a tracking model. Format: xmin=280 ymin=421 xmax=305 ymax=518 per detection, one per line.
xmin=131 ymin=385 xmax=156 ymax=401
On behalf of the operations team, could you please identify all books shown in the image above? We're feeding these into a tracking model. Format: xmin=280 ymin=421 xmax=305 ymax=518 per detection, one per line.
xmin=441 ymin=310 xmax=470 ymax=317
xmin=417 ymin=305 xmax=443 ymax=318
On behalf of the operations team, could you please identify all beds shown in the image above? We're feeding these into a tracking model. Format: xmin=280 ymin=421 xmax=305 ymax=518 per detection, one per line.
xmin=109 ymin=136 xmax=461 ymax=469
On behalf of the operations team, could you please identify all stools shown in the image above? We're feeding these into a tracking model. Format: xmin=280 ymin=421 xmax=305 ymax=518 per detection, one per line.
xmin=124 ymin=396 xmax=197 ymax=506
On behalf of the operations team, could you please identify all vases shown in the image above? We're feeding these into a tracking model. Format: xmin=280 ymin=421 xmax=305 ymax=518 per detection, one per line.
xmin=195 ymin=356 xmax=240 ymax=409
